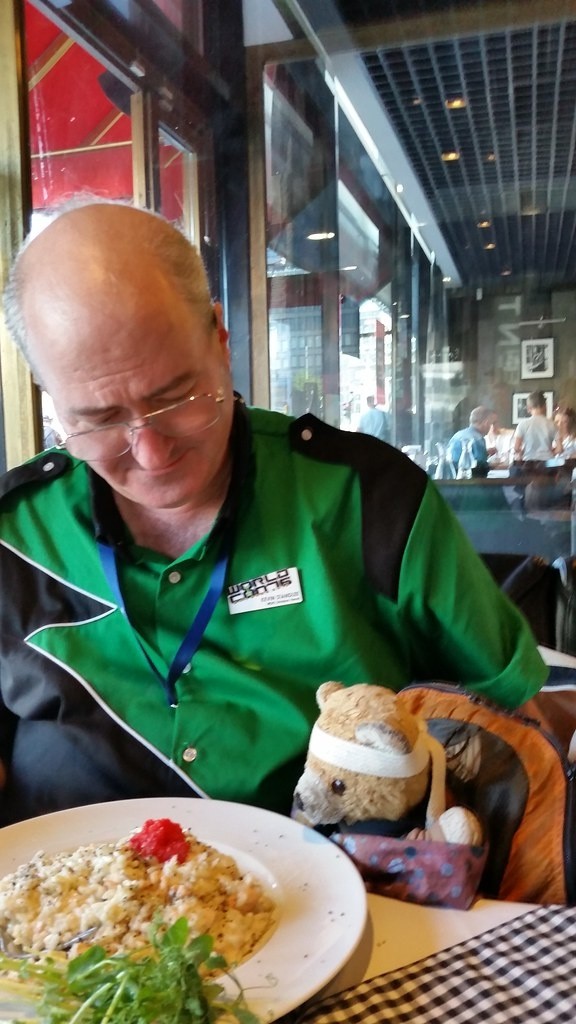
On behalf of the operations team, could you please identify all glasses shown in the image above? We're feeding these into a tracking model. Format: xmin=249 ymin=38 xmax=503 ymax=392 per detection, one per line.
xmin=46 ymin=307 xmax=226 ymax=461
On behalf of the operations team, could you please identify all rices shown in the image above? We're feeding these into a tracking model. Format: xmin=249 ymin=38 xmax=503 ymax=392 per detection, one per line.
xmin=0 ymin=828 xmax=268 ymax=977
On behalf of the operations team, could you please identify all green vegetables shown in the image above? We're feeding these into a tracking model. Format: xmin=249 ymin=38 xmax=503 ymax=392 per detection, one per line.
xmin=0 ymin=906 xmax=262 ymax=1023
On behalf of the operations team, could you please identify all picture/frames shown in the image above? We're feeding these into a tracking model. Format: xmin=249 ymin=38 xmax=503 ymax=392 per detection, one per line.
xmin=520 ymin=336 xmax=556 ymax=381
xmin=511 ymin=390 xmax=555 ymax=425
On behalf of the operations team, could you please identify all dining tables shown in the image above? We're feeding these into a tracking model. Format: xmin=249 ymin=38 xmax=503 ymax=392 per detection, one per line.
xmin=293 ymin=892 xmax=576 ymax=1024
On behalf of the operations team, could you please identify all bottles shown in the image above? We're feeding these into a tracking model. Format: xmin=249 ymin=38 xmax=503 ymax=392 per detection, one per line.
xmin=432 ymin=443 xmax=457 ymax=480
xmin=457 ymin=441 xmax=473 ymax=480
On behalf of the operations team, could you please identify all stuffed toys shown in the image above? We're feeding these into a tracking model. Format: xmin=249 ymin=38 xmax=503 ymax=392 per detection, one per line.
xmin=293 ymin=677 xmax=483 ymax=854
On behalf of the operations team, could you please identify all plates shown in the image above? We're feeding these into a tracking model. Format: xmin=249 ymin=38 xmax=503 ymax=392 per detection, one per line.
xmin=0 ymin=796 xmax=367 ymax=1023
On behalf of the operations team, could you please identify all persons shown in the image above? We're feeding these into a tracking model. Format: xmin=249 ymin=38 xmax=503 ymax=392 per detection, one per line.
xmin=358 ymin=396 xmax=390 ymax=440
xmin=447 ymin=390 xmax=576 ymax=479
xmin=2 ymin=204 xmax=556 ymax=836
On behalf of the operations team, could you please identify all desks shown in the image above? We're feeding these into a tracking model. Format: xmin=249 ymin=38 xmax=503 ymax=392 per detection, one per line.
xmin=431 ymin=470 xmax=576 ymax=509
xmin=450 ymin=511 xmax=576 ymax=561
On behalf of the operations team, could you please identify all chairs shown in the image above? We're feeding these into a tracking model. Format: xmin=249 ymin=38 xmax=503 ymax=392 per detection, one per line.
xmin=534 ymin=684 xmax=576 ymax=752
